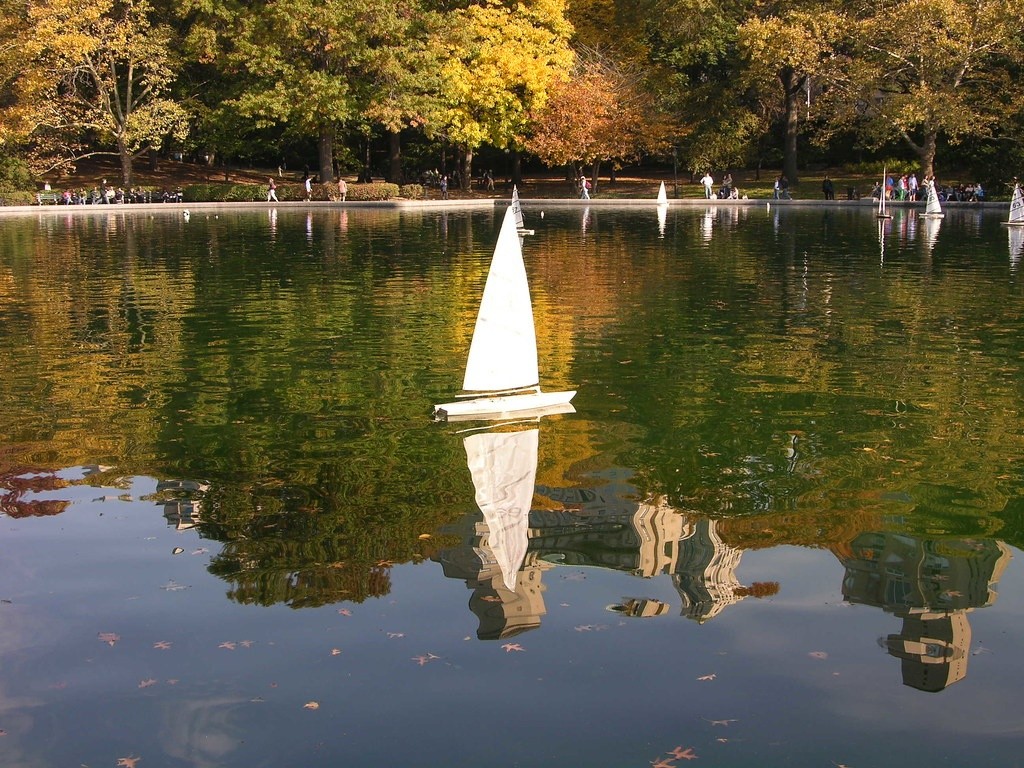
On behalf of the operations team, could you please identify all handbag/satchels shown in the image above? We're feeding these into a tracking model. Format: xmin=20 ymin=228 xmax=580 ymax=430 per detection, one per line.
xmin=177 ymin=192 xmax=183 ymax=196
xmin=271 ymin=185 xmax=276 ymax=189
xmin=886 ymin=185 xmax=891 ymax=190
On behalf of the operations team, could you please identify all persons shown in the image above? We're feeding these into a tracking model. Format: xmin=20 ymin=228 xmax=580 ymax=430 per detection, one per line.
xmin=45 ymin=146 xmax=348 ymax=205
xmin=701 ymin=170 xmax=984 ymax=202
xmin=576 ymin=166 xmax=591 ymax=199
xmin=416 ymin=169 xmax=497 ymax=201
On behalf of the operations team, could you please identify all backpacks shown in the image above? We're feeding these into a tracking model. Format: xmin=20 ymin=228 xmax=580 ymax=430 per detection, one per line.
xmin=586 ymin=181 xmax=592 ymax=189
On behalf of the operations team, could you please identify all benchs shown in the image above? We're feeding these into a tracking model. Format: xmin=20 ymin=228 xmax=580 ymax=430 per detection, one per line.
xmin=37 ymin=190 xmax=179 ymax=206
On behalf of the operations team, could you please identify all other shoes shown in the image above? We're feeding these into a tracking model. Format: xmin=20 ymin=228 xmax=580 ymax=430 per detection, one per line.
xmin=790 ymin=198 xmax=792 ymax=201
xmin=969 ymin=199 xmax=972 ymax=201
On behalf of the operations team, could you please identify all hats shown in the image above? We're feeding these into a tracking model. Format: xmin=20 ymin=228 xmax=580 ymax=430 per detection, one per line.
xmin=581 ymin=176 xmax=585 ymax=180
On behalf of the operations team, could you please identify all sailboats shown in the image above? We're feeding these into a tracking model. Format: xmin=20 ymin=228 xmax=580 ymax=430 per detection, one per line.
xmin=431 ymin=205 xmax=578 ymax=416
xmin=511 ymin=185 xmax=535 ymax=237
xmin=1001 ymin=226 xmax=1024 ymax=291
xmin=434 ymin=403 xmax=578 ymax=594
xmin=998 ymin=183 xmax=1024 ymax=226
xmin=918 ymin=216 xmax=945 ymax=257
xmin=656 ymin=180 xmax=670 ymax=206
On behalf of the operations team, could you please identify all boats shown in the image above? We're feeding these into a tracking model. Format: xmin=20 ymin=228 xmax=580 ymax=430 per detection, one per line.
xmin=918 ymin=179 xmax=945 ymax=219
xmin=876 ymin=167 xmax=894 ymax=219
